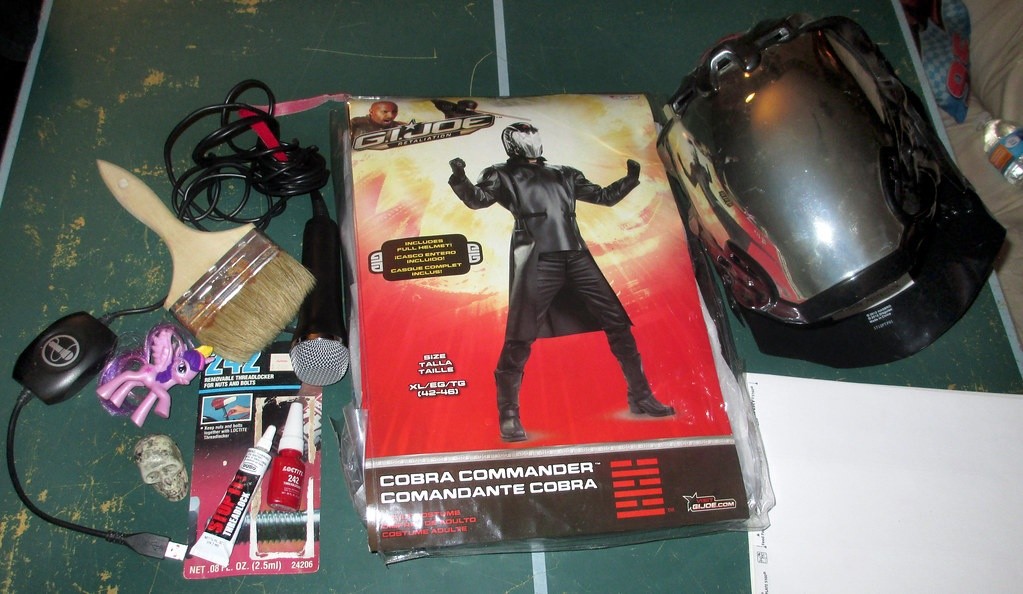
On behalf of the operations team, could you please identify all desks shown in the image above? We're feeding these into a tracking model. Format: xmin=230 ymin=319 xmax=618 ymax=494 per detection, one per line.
xmin=0 ymin=0 xmax=1023 ymax=594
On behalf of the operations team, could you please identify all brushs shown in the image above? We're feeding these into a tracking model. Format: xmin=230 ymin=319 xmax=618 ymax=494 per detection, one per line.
xmin=96 ymin=158 xmax=317 ymax=364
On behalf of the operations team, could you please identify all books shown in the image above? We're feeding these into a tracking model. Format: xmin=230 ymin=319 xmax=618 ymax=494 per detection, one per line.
xmin=330 ymin=92 xmax=750 ymax=551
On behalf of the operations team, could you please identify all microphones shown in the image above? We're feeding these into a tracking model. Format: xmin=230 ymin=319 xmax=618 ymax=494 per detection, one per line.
xmin=287 ymin=216 xmax=351 ymax=386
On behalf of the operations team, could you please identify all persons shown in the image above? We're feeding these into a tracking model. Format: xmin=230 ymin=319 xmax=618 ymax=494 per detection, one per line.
xmin=447 ymin=122 xmax=674 ymax=441
xmin=350 ymin=101 xmax=406 ymax=141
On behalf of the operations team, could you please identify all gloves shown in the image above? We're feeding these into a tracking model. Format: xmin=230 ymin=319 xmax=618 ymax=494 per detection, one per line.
xmin=626 ymin=159 xmax=640 ymax=179
xmin=449 ymin=157 xmax=467 ymax=178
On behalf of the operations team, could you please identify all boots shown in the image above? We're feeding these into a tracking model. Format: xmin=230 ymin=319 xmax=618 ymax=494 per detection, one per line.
xmin=493 ymin=368 xmax=526 ymax=441
xmin=617 ymin=353 xmax=675 ymax=417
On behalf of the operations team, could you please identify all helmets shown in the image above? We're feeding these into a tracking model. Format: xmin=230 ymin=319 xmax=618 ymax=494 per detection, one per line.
xmin=502 ymin=122 xmax=542 ymax=158
xmin=654 ymin=11 xmax=1008 ymax=370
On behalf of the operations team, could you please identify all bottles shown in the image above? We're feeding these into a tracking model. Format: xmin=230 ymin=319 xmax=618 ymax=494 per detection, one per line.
xmin=974 ymin=107 xmax=1023 ymax=190
